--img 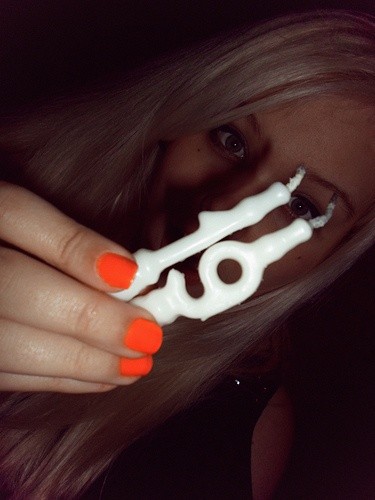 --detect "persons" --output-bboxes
[0,0,375,500]
